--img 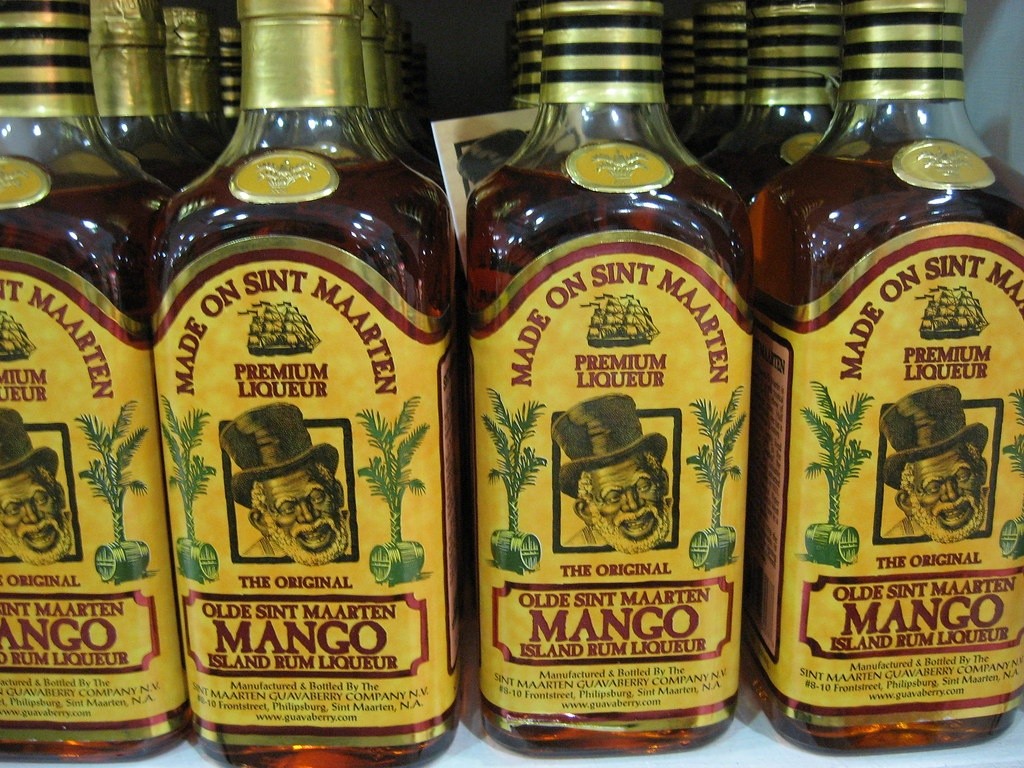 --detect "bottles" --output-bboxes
[0,2,242,762]
[663,3,1024,753]
[465,0,758,759]
[148,0,471,768]
